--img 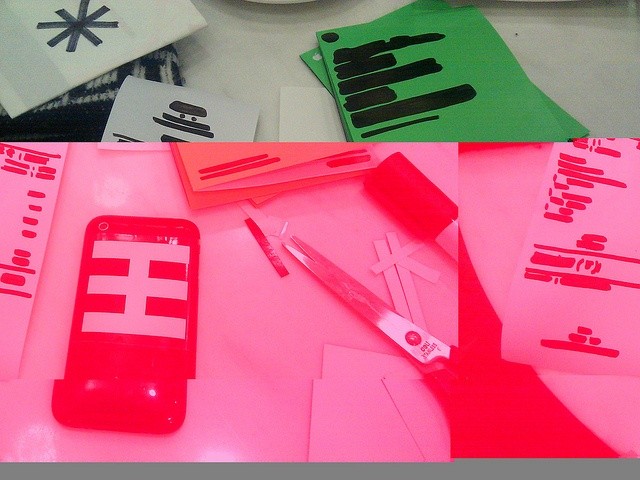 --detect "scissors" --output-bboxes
[282,235,622,459]
